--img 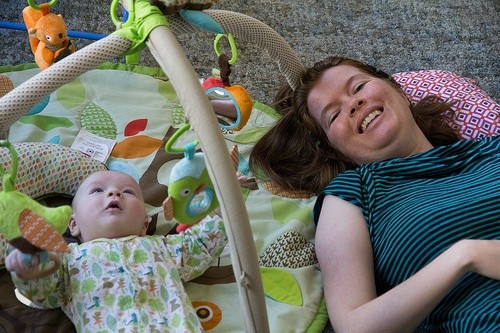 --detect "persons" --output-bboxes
[4,168,235,332]
[246,54,500,333]
